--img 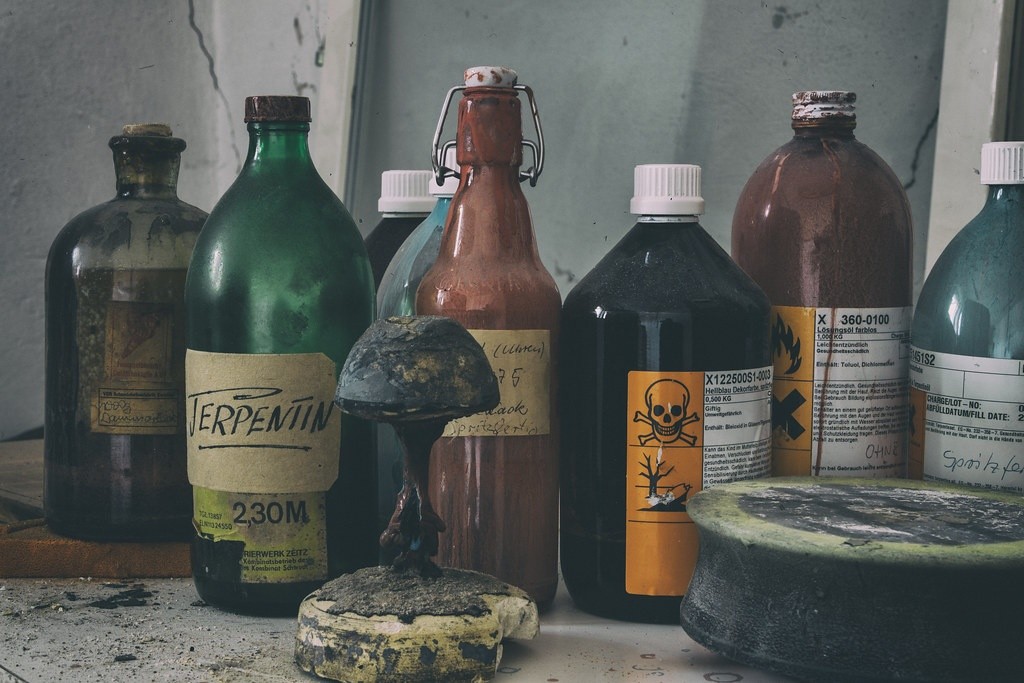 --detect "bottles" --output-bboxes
[365,170,438,294]
[415,67,561,608]
[733,91,914,479]
[45,125,209,540]
[907,143,1024,494]
[374,150,464,540]
[185,96,373,619]
[561,163,773,624]
